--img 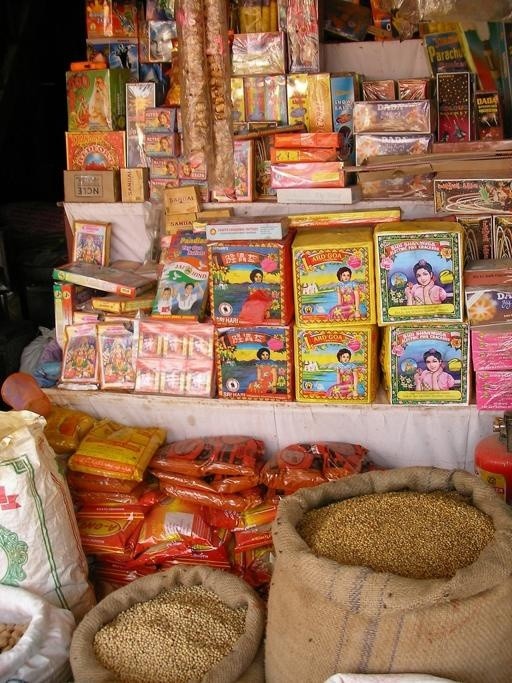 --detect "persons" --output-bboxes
[411,174,423,192]
[156,111,192,190]
[150,23,177,61]
